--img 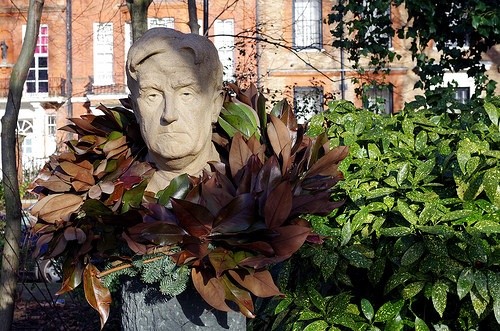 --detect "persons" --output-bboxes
[122,25,227,207]
